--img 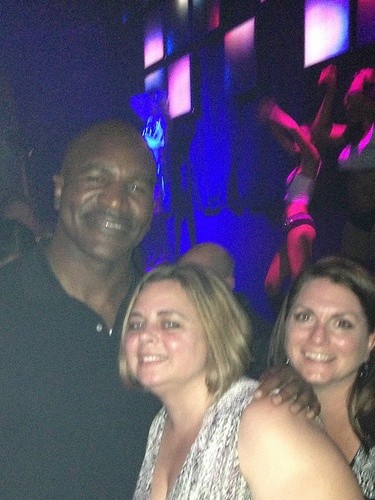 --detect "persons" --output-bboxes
[118,263,365,500]
[0,117,322,500]
[0,64,375,379]
[261,254,375,499]
